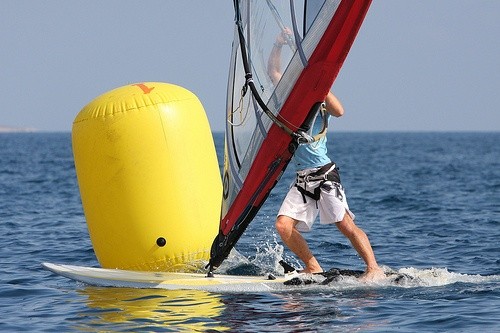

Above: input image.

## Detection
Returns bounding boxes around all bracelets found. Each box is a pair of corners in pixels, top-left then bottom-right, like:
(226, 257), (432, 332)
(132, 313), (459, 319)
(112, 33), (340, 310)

(272, 40), (283, 49)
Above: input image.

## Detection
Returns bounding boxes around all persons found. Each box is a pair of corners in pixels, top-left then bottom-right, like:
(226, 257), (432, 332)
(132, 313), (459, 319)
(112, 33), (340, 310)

(266, 27), (387, 282)
